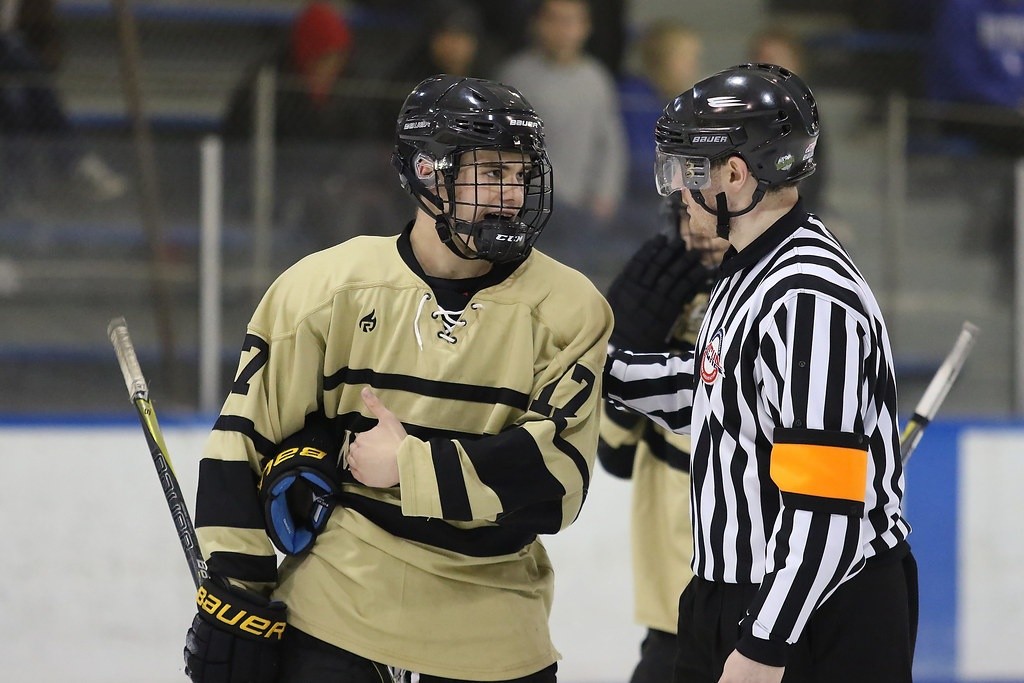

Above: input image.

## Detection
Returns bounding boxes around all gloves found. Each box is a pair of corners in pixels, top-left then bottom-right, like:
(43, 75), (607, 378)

(185, 580), (286, 682)
(259, 408), (349, 556)
(605, 233), (705, 352)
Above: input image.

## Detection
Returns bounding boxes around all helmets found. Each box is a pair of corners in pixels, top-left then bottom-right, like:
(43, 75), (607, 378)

(392, 72), (553, 264)
(653, 61), (820, 188)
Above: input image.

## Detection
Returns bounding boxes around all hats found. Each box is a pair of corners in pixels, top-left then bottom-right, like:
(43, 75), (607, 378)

(294, 4), (353, 68)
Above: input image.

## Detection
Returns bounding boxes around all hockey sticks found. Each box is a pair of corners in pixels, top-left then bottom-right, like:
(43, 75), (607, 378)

(900, 322), (983, 465)
(107, 316), (210, 594)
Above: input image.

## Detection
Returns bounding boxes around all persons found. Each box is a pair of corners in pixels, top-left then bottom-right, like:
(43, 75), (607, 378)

(215, 0), (396, 141)
(379, 0), (486, 140)
(750, 28), (855, 252)
(182, 73), (617, 683)
(600, 64), (923, 683)
(931, 0), (1024, 158)
(491, 0), (658, 252)
(609, 18), (708, 207)
